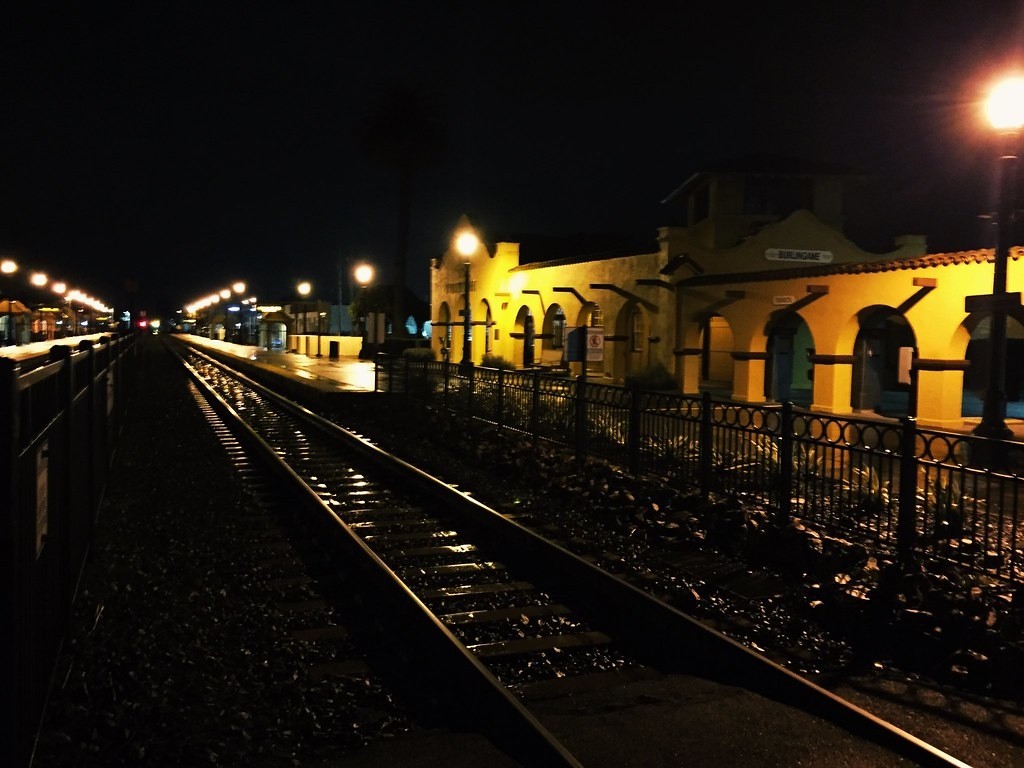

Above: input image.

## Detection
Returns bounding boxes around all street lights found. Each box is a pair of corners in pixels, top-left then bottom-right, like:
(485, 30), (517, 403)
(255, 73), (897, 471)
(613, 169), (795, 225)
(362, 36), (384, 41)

(455, 233), (480, 377)
(968, 60), (1024, 467)
(30, 272), (48, 321)
(0, 258), (20, 346)
(353, 263), (376, 338)
